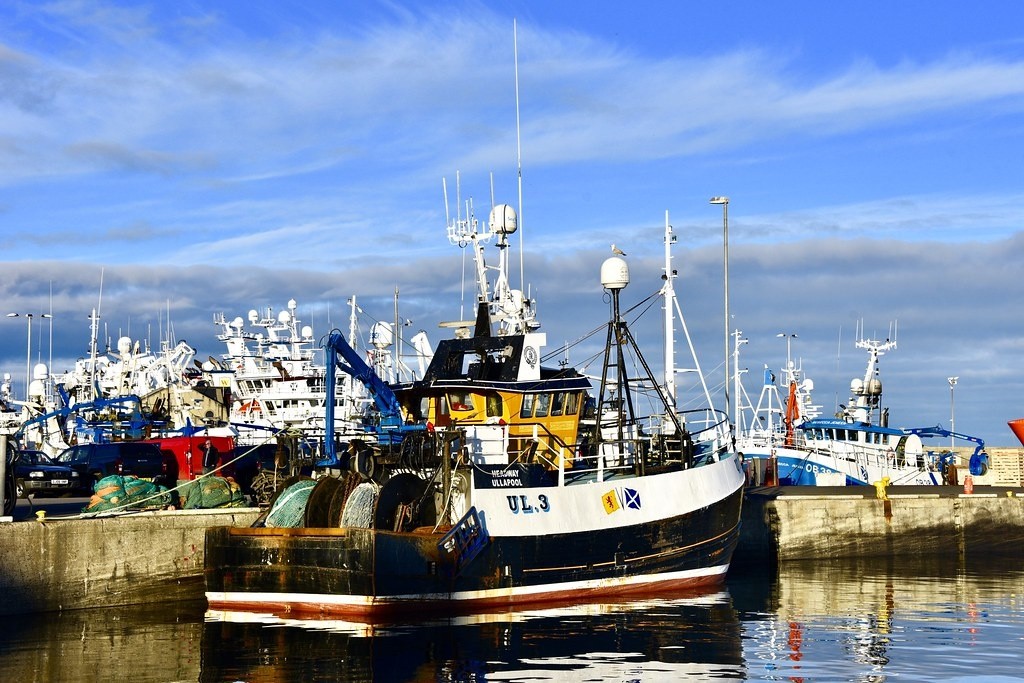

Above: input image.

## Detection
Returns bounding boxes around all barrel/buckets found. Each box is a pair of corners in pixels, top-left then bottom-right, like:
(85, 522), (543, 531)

(964, 475), (973, 494)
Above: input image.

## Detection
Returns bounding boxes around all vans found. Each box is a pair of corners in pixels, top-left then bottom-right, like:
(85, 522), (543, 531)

(49, 441), (170, 493)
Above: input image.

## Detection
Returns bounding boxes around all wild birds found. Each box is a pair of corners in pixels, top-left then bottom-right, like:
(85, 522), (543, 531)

(610, 243), (627, 257)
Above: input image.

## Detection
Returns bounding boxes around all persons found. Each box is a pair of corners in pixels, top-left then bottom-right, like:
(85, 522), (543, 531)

(197, 440), (220, 478)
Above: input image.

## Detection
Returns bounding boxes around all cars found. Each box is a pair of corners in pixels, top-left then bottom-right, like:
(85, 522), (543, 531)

(7, 450), (82, 498)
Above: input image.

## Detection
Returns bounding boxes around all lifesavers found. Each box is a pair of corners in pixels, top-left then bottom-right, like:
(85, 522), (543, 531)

(886, 448), (895, 462)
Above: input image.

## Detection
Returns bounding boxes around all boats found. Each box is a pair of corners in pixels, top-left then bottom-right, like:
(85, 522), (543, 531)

(0, 268), (436, 482)
(686, 314), (991, 491)
(200, 19), (754, 635)
